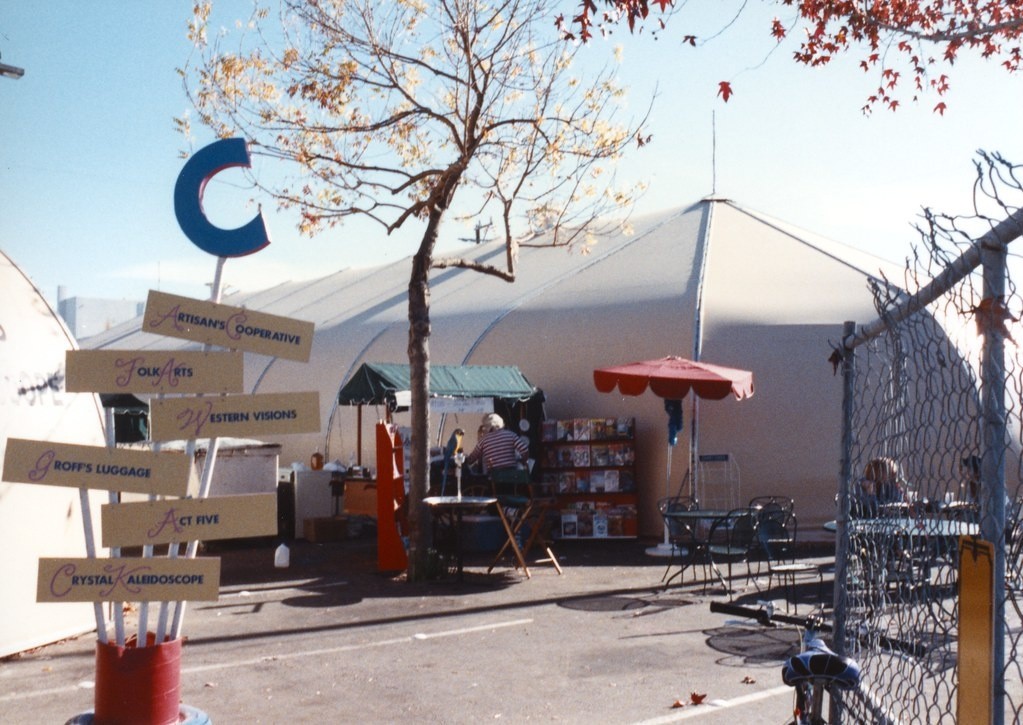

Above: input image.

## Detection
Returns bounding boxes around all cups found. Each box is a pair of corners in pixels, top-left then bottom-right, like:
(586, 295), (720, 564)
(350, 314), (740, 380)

(945, 492), (954, 504)
(907, 491), (918, 504)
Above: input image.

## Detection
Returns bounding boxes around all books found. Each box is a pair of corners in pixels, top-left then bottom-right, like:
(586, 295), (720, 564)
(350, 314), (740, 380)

(545, 417), (638, 536)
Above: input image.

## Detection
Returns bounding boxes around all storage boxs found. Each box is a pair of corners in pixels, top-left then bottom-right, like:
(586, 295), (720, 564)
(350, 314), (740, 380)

(303, 515), (348, 544)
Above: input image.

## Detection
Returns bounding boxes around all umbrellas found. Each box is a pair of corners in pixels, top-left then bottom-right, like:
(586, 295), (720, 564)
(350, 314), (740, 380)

(592, 356), (753, 554)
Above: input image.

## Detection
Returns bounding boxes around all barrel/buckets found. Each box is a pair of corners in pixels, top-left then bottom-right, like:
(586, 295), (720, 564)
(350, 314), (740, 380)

(94, 630), (182, 725)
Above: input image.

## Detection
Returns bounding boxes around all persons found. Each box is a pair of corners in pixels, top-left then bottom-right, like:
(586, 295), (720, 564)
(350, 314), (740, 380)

(936, 454), (1012, 556)
(465, 412), (542, 556)
(853, 457), (911, 566)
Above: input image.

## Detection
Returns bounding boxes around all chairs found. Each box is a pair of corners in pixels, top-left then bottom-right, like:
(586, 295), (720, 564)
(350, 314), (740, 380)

(487, 457), (562, 580)
(656, 491), (859, 616)
(452, 485), (493, 516)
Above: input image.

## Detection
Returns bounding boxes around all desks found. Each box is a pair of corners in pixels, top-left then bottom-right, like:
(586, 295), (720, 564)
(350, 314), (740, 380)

(826, 499), (981, 635)
(422, 495), (498, 591)
(662, 510), (758, 596)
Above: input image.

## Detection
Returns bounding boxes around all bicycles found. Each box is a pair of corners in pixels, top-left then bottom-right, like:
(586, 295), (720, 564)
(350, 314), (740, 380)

(709, 599), (927, 725)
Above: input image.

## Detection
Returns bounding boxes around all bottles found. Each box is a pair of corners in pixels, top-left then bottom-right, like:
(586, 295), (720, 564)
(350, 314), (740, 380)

(311, 447), (323, 471)
(274, 542), (290, 568)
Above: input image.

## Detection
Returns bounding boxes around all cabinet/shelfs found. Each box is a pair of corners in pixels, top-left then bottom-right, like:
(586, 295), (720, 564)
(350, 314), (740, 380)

(536, 416), (640, 540)
